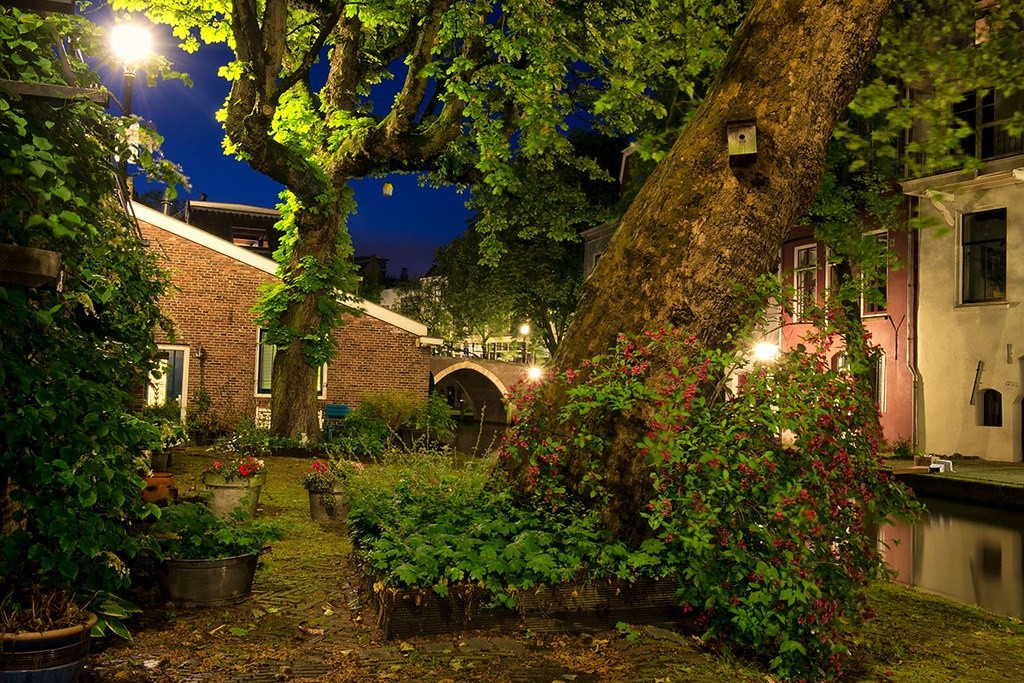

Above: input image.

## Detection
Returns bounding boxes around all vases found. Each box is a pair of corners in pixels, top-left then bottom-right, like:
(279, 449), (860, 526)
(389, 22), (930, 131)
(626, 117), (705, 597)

(307, 485), (352, 525)
(203, 472), (266, 520)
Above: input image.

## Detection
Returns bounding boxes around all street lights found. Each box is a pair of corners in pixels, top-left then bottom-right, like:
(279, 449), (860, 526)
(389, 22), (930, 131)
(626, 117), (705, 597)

(107, 23), (153, 201)
(520, 324), (529, 364)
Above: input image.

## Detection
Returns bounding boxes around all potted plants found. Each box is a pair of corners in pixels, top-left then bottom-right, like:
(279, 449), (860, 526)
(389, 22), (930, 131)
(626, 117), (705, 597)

(0, 490), (296, 683)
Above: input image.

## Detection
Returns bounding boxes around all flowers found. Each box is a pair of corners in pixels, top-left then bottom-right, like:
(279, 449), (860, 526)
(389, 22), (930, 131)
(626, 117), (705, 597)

(204, 453), (266, 484)
(304, 460), (364, 516)
